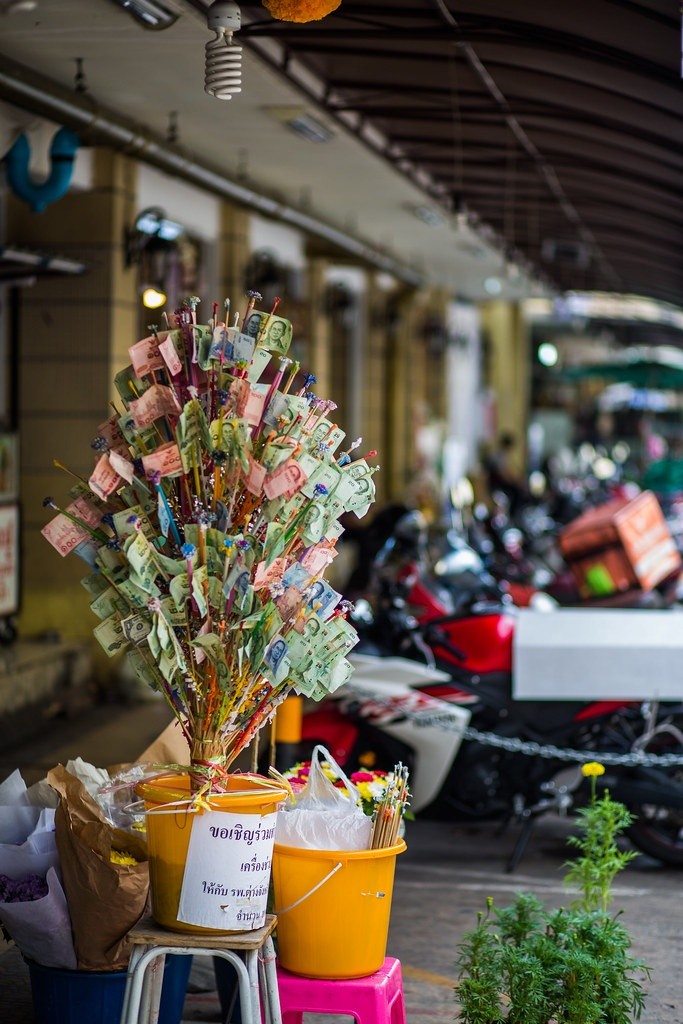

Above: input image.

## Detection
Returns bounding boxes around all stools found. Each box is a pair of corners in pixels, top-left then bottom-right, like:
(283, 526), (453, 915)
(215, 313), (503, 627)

(259, 956), (406, 1024)
(123, 911), (283, 1024)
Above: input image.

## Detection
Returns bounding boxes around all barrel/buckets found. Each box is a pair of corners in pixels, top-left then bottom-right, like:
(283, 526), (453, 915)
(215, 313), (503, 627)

(271, 838), (407, 981)
(120, 775), (288, 936)
(17, 950), (192, 1023)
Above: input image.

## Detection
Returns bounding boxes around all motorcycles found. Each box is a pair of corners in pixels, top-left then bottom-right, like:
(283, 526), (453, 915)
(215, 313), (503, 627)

(267, 427), (682, 876)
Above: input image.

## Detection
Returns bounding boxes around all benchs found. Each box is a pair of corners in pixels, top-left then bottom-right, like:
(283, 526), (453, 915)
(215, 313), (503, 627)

(276, 701), (359, 775)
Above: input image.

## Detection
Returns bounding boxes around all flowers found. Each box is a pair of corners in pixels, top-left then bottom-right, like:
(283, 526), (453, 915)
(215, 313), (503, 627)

(564, 763), (640, 912)
(276, 751), (423, 834)
